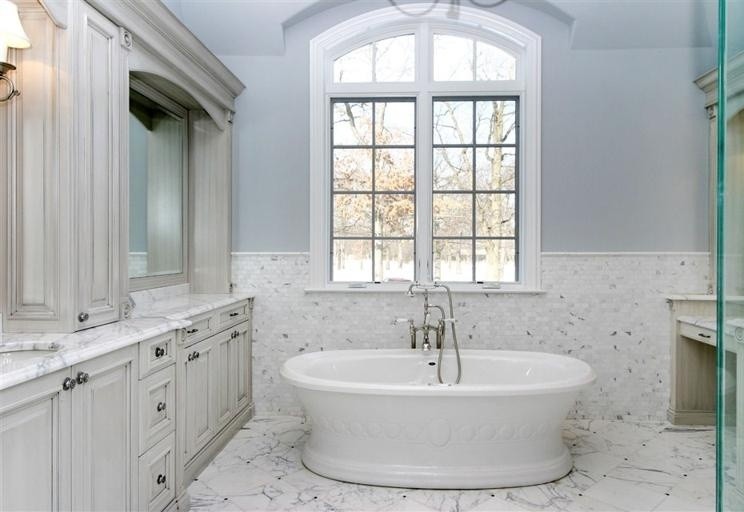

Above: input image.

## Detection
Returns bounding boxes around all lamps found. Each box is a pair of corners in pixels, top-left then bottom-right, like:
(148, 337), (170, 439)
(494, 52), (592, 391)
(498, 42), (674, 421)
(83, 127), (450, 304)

(1, 1), (33, 102)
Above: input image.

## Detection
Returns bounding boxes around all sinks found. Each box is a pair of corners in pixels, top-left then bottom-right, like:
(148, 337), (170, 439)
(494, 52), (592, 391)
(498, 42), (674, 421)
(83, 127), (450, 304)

(0, 343), (63, 374)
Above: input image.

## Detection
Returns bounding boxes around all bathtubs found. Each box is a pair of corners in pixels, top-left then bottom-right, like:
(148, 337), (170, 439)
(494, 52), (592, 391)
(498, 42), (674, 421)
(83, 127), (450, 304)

(278, 350), (597, 491)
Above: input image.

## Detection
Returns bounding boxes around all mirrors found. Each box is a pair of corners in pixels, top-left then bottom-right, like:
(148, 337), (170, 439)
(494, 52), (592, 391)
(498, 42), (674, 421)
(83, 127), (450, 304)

(128, 84), (186, 278)
